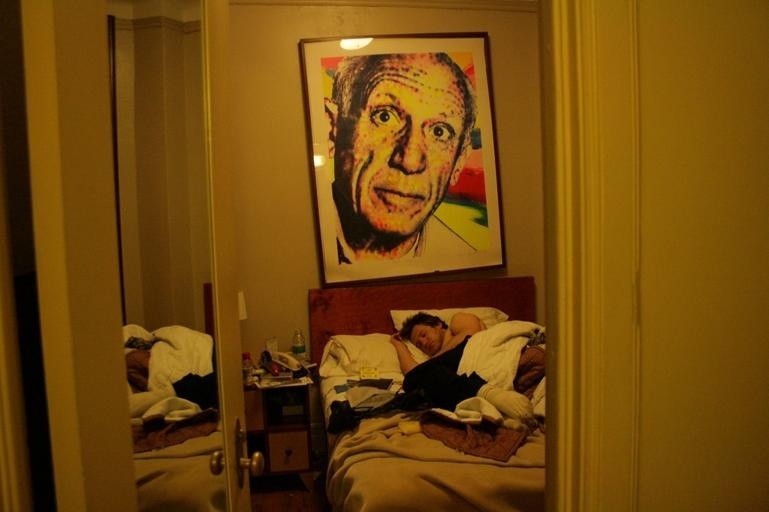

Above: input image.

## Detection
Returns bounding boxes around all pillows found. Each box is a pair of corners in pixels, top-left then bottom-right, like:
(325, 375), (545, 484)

(390, 306), (510, 365)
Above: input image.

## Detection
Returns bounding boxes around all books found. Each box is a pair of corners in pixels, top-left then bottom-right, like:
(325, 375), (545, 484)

(349, 376), (394, 391)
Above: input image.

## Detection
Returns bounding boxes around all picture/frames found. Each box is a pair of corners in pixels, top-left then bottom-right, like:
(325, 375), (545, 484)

(298, 31), (507, 290)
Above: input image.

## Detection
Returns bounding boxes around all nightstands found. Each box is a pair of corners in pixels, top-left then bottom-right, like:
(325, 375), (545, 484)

(244, 372), (325, 489)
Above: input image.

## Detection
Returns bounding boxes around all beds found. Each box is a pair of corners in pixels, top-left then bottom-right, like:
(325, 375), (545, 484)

(304, 278), (549, 512)
(121, 283), (232, 512)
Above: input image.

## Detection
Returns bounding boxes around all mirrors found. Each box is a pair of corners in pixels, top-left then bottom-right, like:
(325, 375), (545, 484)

(18, 0), (251, 512)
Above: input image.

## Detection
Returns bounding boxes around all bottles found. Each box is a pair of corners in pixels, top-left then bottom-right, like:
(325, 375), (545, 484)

(241, 352), (254, 391)
(291, 329), (306, 360)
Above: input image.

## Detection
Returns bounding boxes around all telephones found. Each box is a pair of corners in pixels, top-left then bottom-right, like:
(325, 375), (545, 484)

(271, 351), (307, 371)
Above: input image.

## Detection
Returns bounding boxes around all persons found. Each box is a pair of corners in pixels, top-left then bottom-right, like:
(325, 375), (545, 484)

(390, 311), (489, 375)
(322, 53), (478, 266)
